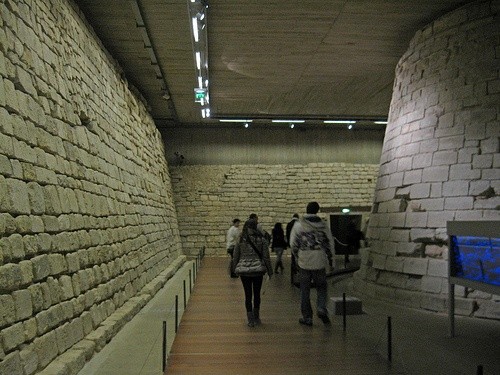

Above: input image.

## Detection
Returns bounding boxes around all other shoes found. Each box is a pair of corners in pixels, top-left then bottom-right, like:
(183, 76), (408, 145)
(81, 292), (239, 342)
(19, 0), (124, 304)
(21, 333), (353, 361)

(254, 317), (261, 322)
(317, 312), (331, 325)
(248, 322), (253, 326)
(274, 271), (279, 273)
(299, 318), (314, 326)
(281, 267), (285, 270)
(230, 274), (239, 278)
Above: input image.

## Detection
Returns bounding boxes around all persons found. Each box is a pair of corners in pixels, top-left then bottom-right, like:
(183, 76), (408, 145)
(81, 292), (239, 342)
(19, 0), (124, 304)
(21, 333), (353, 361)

(290, 201), (336, 328)
(230, 219), (274, 327)
(226, 213), (370, 278)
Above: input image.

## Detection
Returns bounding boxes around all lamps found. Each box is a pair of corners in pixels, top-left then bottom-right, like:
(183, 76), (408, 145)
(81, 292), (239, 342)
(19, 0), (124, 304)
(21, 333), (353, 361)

(186, 0), (211, 119)
